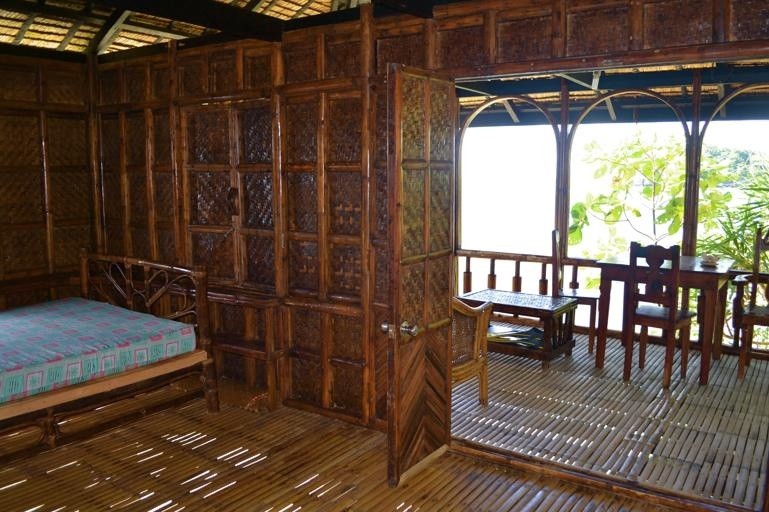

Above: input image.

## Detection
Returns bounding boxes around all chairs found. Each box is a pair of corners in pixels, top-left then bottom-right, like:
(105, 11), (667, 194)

(450, 297), (492, 407)
(552, 230), (599, 354)
(733, 228), (769, 380)
(625, 241), (697, 389)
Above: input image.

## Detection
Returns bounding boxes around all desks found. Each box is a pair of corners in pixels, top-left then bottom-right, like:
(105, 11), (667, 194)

(593, 252), (736, 388)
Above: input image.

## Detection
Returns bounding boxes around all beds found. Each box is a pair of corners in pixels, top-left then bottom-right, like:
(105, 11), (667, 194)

(1, 250), (221, 457)
